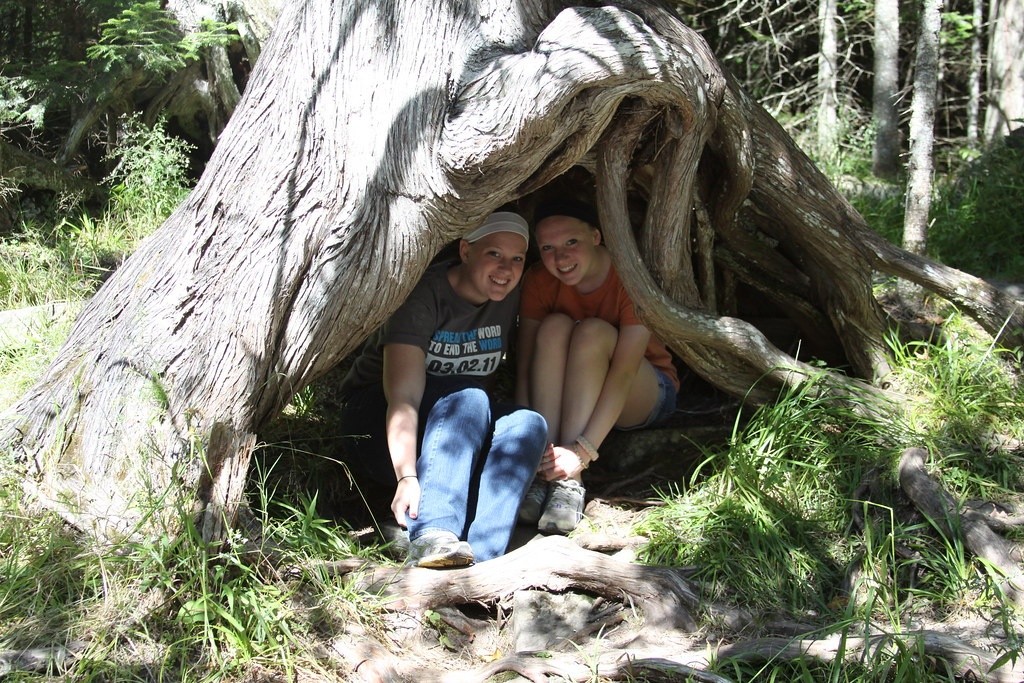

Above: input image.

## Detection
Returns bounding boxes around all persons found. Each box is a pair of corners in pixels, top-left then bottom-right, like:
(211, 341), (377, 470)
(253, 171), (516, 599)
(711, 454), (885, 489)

(511, 197), (682, 536)
(335, 202), (551, 571)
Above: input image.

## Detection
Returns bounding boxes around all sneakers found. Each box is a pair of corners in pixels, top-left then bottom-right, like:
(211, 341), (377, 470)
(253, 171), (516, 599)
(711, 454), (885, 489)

(408, 530), (474, 568)
(517, 475), (586, 535)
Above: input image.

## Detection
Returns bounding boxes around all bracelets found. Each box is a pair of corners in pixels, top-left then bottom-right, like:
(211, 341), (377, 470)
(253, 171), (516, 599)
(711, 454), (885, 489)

(397, 473), (417, 483)
(573, 442), (590, 469)
(576, 435), (599, 462)
